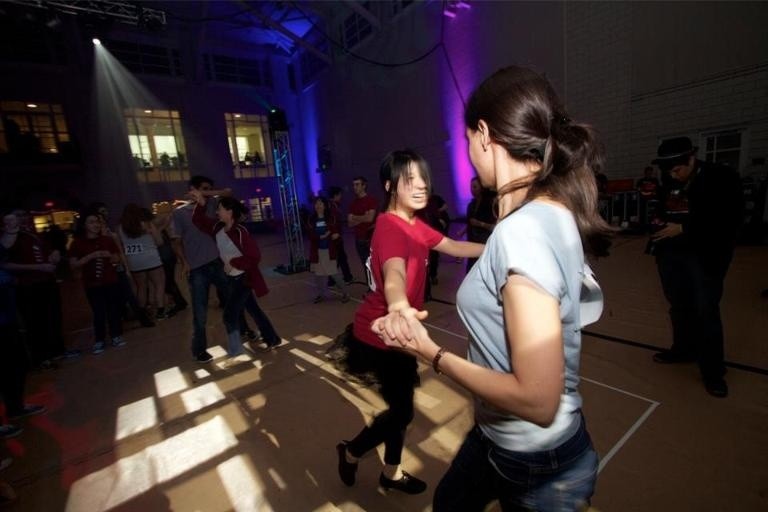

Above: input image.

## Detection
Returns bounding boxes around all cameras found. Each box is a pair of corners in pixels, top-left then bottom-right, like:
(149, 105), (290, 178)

(95, 251), (105, 257)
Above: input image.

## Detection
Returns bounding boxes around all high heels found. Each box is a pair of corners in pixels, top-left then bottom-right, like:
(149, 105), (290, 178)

(335, 438), (360, 488)
(379, 466), (428, 495)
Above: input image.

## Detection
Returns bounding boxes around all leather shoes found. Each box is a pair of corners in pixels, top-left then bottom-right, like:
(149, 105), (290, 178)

(702, 377), (728, 398)
(652, 351), (667, 364)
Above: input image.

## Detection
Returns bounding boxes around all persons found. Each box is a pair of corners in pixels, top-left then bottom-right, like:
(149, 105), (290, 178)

(1, 203), (190, 441)
(306, 176), (382, 303)
(169, 176), (281, 364)
(636, 138), (742, 399)
(413, 173), (611, 310)
(366, 64), (620, 512)
(337, 148), (497, 497)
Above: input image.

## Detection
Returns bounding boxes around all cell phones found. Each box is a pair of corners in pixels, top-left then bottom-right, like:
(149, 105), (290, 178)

(647, 224), (668, 234)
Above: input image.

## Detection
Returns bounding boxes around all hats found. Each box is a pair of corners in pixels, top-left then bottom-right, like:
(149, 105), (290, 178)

(650, 136), (698, 164)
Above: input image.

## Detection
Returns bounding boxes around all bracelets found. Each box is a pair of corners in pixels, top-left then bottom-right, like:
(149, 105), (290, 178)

(432, 346), (450, 376)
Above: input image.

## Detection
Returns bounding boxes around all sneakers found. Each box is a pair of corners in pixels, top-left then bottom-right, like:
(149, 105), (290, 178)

(8, 403), (47, 420)
(39, 360), (58, 370)
(242, 328), (257, 342)
(51, 348), (81, 361)
(0, 424), (24, 440)
(111, 336), (127, 347)
(92, 341), (104, 354)
(191, 350), (213, 363)
